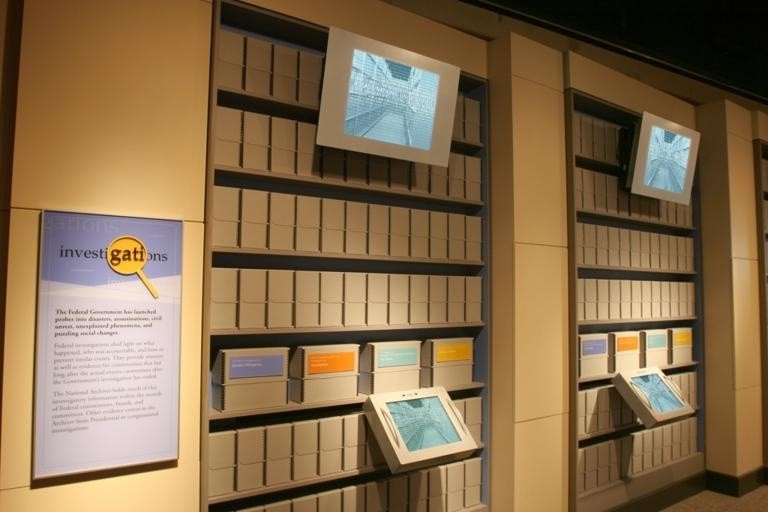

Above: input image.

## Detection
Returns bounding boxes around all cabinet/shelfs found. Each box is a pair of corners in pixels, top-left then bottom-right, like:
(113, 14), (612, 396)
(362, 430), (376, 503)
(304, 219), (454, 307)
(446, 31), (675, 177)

(563, 48), (709, 512)
(197, 0), (491, 510)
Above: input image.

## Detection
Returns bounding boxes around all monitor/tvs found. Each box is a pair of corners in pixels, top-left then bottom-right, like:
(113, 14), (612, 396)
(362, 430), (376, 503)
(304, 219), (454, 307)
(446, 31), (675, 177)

(363, 386), (478, 474)
(315, 25), (461, 167)
(611, 365), (695, 429)
(626, 111), (701, 206)
